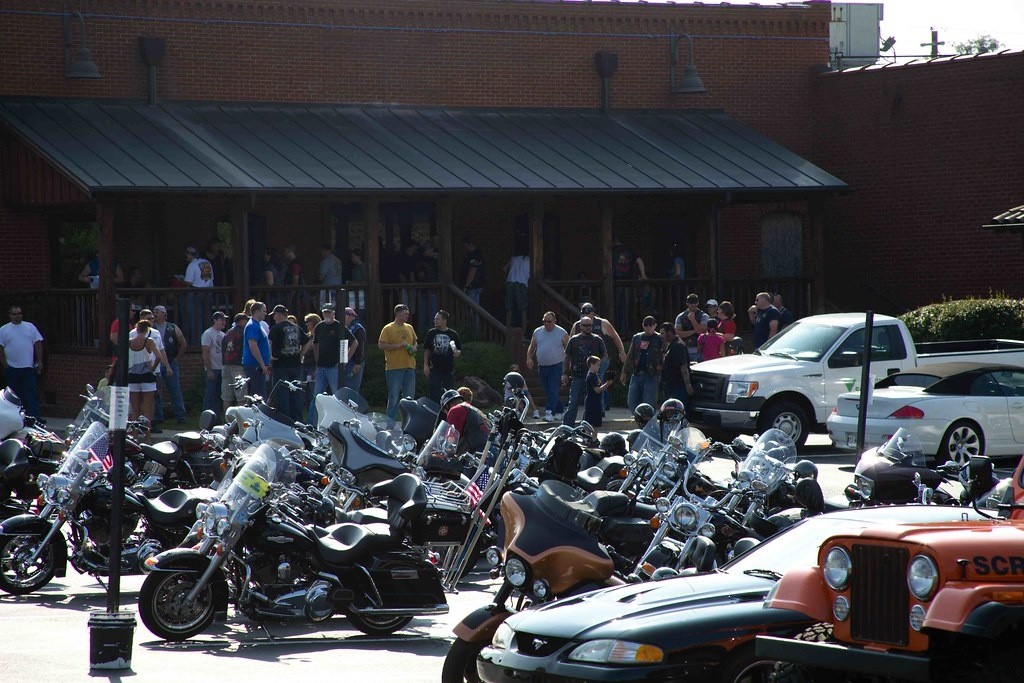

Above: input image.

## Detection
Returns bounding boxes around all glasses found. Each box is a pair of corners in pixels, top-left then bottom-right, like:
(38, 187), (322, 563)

(757, 297), (761, 301)
(643, 324), (653, 327)
(687, 301), (698, 304)
(543, 319), (555, 323)
(581, 323), (593, 327)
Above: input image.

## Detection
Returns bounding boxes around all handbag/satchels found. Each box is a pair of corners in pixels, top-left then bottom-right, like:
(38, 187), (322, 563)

(697, 345), (703, 362)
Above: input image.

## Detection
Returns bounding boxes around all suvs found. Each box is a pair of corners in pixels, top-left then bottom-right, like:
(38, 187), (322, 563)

(751, 461), (1024, 683)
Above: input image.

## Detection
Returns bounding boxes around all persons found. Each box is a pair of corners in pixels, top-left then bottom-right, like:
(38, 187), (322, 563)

(201, 299), (366, 432)
(501, 232), (694, 343)
(377, 303), (419, 430)
(0, 306), (47, 424)
(80, 234), (486, 348)
(96, 300), (187, 446)
(433, 387), (492, 458)
(423, 309), (462, 404)
(504, 292), (793, 446)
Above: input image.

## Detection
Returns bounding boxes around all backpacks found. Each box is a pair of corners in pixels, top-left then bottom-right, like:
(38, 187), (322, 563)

(460, 404), (493, 448)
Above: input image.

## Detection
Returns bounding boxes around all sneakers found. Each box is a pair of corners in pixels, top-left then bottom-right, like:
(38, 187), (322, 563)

(554, 413), (563, 420)
(533, 410), (539, 418)
(544, 410), (553, 421)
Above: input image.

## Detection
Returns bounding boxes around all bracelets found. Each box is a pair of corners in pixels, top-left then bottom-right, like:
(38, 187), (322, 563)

(462, 284), (469, 289)
(165, 365), (171, 370)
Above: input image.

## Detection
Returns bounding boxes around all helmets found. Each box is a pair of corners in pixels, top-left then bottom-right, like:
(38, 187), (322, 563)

(598, 431), (626, 453)
(633, 403), (656, 423)
(657, 398), (686, 423)
(441, 388), (463, 405)
(504, 372), (525, 391)
(626, 429), (644, 446)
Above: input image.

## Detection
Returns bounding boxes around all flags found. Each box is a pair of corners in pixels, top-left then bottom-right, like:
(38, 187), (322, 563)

(84, 433), (114, 478)
(463, 464), (495, 526)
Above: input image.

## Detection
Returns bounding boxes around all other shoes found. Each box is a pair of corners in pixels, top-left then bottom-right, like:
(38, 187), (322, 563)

(151, 426), (162, 433)
(37, 418), (47, 425)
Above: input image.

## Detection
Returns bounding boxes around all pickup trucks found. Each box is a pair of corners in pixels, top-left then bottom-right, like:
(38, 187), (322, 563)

(689, 312), (1024, 461)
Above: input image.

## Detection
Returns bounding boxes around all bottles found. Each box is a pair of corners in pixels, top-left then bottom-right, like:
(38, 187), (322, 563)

(405, 344), (416, 356)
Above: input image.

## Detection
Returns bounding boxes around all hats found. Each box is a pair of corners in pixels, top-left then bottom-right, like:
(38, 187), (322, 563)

(720, 301), (734, 315)
(152, 306), (167, 313)
(268, 305), (289, 317)
(345, 308), (357, 318)
(211, 311), (230, 319)
(322, 303), (335, 313)
(706, 299), (719, 306)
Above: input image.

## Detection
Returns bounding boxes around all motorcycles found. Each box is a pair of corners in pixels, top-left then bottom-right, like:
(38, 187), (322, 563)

(1, 374), (1014, 683)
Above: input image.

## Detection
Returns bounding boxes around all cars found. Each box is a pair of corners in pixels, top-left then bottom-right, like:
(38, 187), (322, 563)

(476, 504), (1001, 683)
(825, 361), (1024, 468)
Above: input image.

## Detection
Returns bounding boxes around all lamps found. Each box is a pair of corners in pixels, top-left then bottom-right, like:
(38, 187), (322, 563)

(670, 26), (709, 95)
(63, 7), (103, 79)
